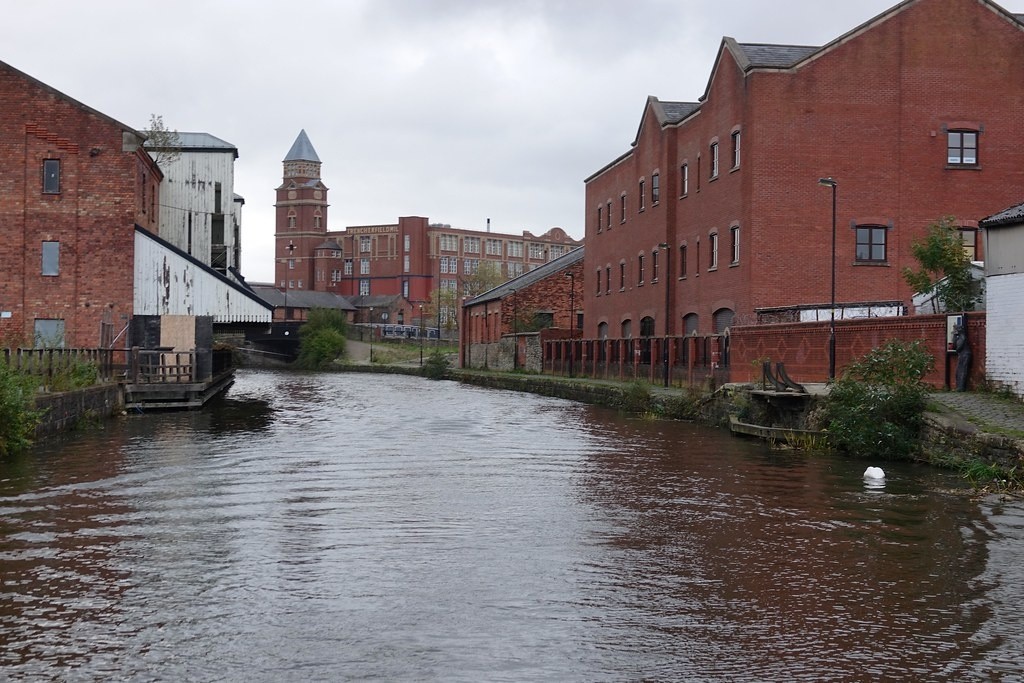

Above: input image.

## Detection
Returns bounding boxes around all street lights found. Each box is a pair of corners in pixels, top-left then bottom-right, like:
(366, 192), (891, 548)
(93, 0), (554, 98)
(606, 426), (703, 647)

(360, 293), (363, 341)
(819, 177), (838, 386)
(509, 288), (517, 369)
(419, 304), (423, 365)
(564, 272), (573, 378)
(369, 306), (373, 362)
